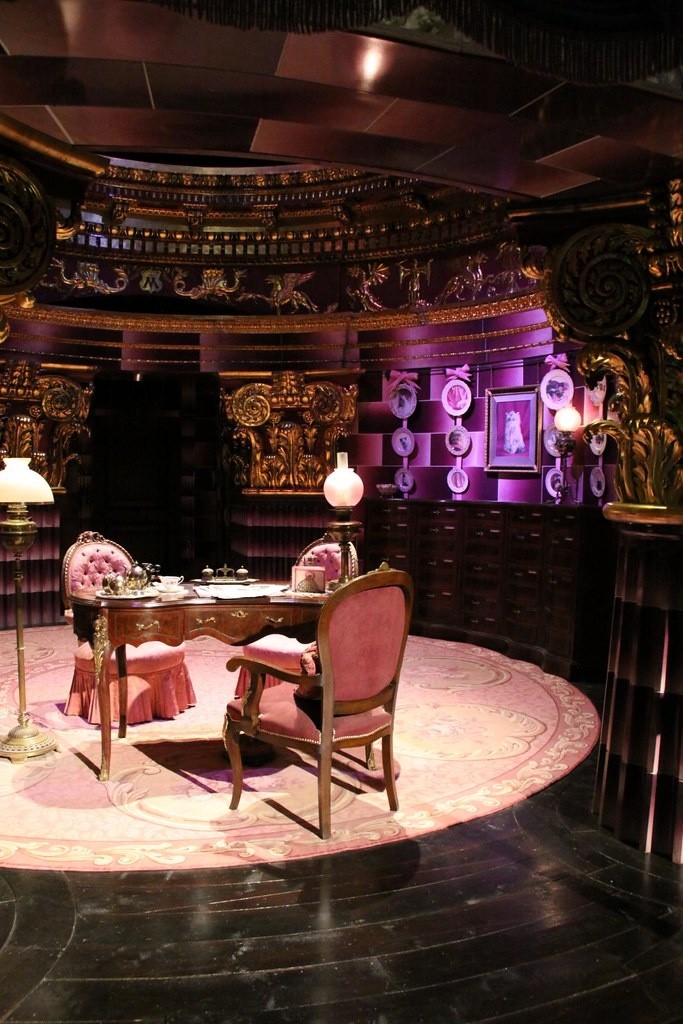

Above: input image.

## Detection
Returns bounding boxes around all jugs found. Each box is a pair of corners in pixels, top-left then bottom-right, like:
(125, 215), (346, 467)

(158, 575), (184, 588)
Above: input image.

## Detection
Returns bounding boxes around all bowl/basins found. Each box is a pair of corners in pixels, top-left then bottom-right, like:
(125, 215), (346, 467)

(376, 483), (399, 494)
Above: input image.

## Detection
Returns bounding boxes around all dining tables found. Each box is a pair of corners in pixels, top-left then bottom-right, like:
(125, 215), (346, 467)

(63, 578), (378, 783)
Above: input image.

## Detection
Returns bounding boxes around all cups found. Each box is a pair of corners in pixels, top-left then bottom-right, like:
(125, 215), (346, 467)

(202, 565), (213, 580)
(236, 566), (248, 580)
(102, 571), (115, 595)
(110, 574), (125, 595)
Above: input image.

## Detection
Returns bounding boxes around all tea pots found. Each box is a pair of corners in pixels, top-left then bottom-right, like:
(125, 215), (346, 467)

(123, 561), (151, 590)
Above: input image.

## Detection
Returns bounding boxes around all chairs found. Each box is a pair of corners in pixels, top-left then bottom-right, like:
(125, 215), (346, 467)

(232, 537), (360, 702)
(60, 531), (197, 728)
(221, 562), (416, 840)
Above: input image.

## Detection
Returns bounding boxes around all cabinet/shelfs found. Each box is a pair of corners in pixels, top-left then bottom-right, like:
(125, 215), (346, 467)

(366, 495), (617, 681)
(229, 498), (363, 581)
(0, 494), (72, 629)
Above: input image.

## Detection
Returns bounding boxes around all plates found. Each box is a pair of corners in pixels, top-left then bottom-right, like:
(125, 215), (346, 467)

(96, 590), (161, 599)
(155, 586), (184, 592)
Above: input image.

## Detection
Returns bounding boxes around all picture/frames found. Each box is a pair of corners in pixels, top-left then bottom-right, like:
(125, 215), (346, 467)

(386, 367), (607, 497)
(484, 384), (545, 477)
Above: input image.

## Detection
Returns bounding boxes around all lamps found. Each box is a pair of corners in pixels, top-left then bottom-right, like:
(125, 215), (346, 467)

(554, 406), (582, 506)
(323, 451), (364, 591)
(0, 456), (55, 764)
